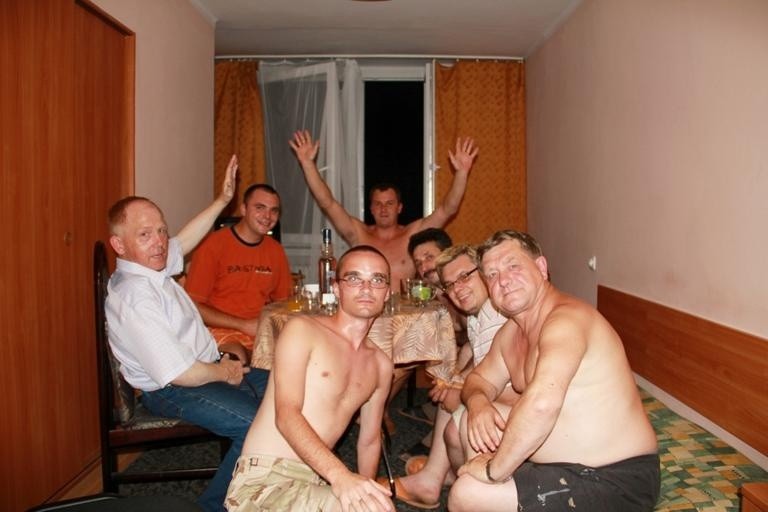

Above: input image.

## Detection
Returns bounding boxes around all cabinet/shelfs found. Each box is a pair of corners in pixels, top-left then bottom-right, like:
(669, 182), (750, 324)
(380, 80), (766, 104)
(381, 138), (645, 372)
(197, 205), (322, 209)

(0, 1), (134, 512)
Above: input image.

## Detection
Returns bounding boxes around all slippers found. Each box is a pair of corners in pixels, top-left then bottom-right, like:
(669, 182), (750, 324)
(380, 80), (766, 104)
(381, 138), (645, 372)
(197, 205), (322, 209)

(398, 441), (431, 462)
(405, 456), (455, 487)
(397, 405), (435, 427)
(376, 475), (441, 510)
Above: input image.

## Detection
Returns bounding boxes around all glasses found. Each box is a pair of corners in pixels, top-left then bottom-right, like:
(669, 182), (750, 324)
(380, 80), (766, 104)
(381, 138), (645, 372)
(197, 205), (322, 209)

(442, 266), (478, 294)
(336, 275), (391, 289)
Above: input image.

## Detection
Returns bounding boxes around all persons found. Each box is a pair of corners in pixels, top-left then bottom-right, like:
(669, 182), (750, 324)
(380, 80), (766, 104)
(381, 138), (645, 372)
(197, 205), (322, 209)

(448, 229), (661, 512)
(396, 227), (468, 462)
(224, 244), (399, 512)
(184, 183), (292, 354)
(379, 242), (510, 511)
(103, 154), (270, 512)
(288, 129), (479, 294)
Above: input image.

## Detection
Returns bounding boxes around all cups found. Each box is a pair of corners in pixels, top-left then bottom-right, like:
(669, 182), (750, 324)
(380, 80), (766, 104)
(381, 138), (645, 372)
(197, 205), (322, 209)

(285, 286), (302, 312)
(399, 276), (416, 307)
(410, 280), (438, 307)
(381, 293), (398, 317)
(302, 289), (318, 316)
(320, 295), (336, 317)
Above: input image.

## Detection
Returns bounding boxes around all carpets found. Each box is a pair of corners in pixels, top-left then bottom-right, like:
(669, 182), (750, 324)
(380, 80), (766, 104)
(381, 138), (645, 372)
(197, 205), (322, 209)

(100, 387), (451, 511)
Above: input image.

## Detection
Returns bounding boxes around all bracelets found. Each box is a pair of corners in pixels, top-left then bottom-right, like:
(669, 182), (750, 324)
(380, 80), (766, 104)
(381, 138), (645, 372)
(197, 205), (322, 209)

(486, 459), (512, 484)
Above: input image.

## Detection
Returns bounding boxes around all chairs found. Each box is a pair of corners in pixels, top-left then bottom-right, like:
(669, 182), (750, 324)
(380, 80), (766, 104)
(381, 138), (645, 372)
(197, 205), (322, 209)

(92, 241), (232, 494)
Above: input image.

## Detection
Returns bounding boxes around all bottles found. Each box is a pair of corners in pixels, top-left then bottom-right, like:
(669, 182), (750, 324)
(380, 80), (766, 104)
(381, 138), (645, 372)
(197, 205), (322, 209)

(318, 228), (338, 305)
